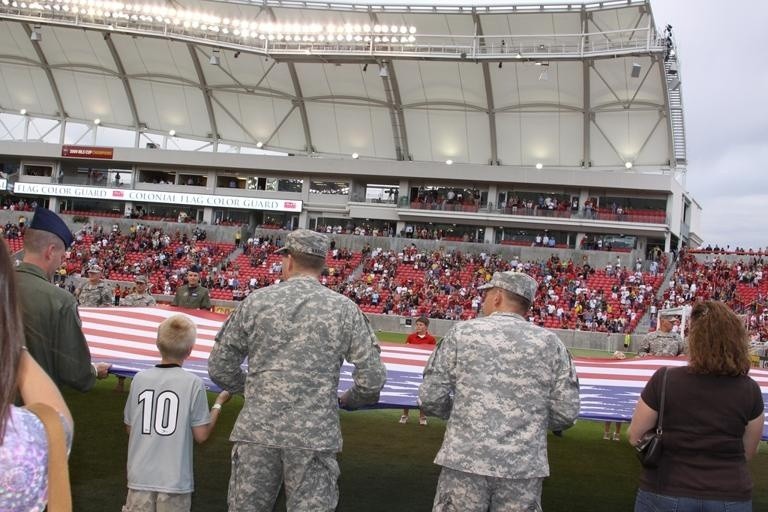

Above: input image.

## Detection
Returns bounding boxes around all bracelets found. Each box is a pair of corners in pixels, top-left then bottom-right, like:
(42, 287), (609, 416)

(211, 403), (222, 410)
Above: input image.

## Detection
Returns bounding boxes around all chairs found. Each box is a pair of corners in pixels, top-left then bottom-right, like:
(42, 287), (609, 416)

(727, 260), (768, 343)
(319, 247), (363, 286)
(3, 234), (24, 254)
(209, 245), (286, 301)
(64, 233), (237, 294)
(612, 246), (632, 253)
(62, 209), (124, 218)
(533, 292), (577, 330)
(587, 269), (665, 334)
(501, 239), (567, 249)
(220, 221), (278, 228)
(359, 261), (477, 320)
(142, 214), (199, 224)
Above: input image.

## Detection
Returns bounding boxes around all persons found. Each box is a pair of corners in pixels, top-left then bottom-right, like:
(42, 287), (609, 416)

(627, 303), (764, 512)
(650, 303), (657, 333)
(309, 221), (395, 238)
(0, 199), (38, 240)
(123, 314), (233, 512)
(657, 303), (693, 309)
(86, 167), (106, 186)
(581, 235), (612, 253)
(732, 309), (768, 342)
(535, 234), (556, 248)
(143, 178), (174, 184)
(208, 231), (387, 512)
(170, 273), (188, 294)
(604, 351), (624, 440)
(112, 206), (293, 231)
(493, 252), (524, 272)
(419, 272), (581, 512)
(405, 224), (485, 243)
(400, 318), (437, 425)
(310, 188), (349, 196)
(410, 185), (480, 212)
(55, 275), (75, 298)
(387, 188), (399, 204)
(12, 204), (110, 512)
(57, 168), (64, 183)
(525, 253), (668, 335)
(170, 266), (211, 313)
(209, 232), (286, 302)
(115, 284), (121, 306)
(52, 221), (208, 274)
(499, 193), (623, 222)
(189, 178), (193, 184)
(230, 180), (236, 187)
(649, 243), (768, 309)
(0, 237), (75, 512)
(319, 238), (493, 320)
(112, 275), (159, 393)
(160, 274), (169, 294)
(638, 309), (688, 358)
(77, 265), (112, 307)
(114, 171), (121, 187)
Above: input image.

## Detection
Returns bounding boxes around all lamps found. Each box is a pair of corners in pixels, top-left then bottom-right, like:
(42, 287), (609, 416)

(379, 68), (386, 77)
(539, 72), (548, 79)
(210, 56), (219, 66)
(632, 61), (640, 78)
(28, 31), (42, 40)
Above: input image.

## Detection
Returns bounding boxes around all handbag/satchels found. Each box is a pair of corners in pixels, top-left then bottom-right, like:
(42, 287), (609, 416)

(634, 365), (670, 471)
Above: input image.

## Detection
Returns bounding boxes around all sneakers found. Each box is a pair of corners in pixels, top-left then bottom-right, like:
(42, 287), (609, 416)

(419, 416), (427, 424)
(613, 432), (619, 441)
(399, 415), (407, 423)
(603, 432), (611, 441)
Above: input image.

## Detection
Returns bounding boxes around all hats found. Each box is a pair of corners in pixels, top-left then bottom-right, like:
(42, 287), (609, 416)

(659, 313), (678, 322)
(272, 228), (330, 261)
(29, 204), (76, 252)
(134, 275), (149, 286)
(476, 271), (540, 304)
(86, 265), (105, 275)
(187, 265), (204, 274)
